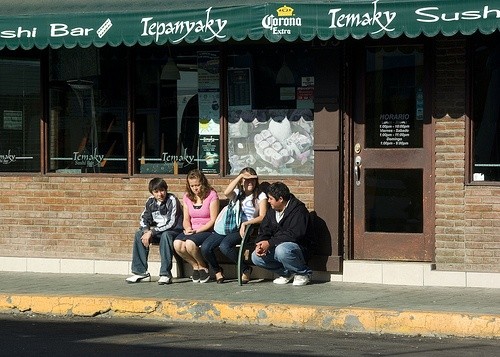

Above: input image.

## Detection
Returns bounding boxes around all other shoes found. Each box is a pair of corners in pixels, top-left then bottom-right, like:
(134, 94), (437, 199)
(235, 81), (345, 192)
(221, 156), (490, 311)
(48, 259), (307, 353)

(273, 273), (294, 284)
(192, 265), (200, 283)
(241, 266), (253, 284)
(214, 267), (224, 283)
(199, 264), (210, 283)
(292, 273), (311, 286)
(125, 273), (151, 283)
(157, 276), (173, 285)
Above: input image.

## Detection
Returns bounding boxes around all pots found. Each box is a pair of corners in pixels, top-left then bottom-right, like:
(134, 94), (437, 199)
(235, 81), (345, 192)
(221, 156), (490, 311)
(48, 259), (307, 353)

(247, 120), (314, 169)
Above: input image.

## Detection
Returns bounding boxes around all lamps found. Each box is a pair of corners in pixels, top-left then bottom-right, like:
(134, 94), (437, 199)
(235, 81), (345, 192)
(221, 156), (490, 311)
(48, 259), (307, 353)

(276, 56), (295, 84)
(160, 45), (180, 80)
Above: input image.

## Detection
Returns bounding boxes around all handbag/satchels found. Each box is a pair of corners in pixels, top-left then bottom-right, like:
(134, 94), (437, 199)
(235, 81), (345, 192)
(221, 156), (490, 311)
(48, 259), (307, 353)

(214, 189), (242, 235)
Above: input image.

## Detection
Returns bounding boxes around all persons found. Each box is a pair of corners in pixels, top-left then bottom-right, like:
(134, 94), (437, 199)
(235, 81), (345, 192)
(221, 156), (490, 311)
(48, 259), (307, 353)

(181, 95), (198, 174)
(173, 170), (220, 283)
(201, 167), (267, 283)
(125, 178), (184, 285)
(251, 182), (313, 286)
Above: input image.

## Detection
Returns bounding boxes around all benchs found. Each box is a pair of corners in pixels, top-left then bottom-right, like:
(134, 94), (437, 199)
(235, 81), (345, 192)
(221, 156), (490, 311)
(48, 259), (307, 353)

(152, 199), (253, 285)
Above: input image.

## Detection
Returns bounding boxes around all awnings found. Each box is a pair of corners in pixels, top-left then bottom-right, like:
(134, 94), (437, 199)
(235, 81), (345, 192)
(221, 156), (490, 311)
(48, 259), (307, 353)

(0, 0), (500, 51)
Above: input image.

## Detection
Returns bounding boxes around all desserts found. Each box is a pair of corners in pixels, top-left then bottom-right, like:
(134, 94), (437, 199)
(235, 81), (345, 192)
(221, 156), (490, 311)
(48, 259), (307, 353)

(254, 130), (290, 167)
